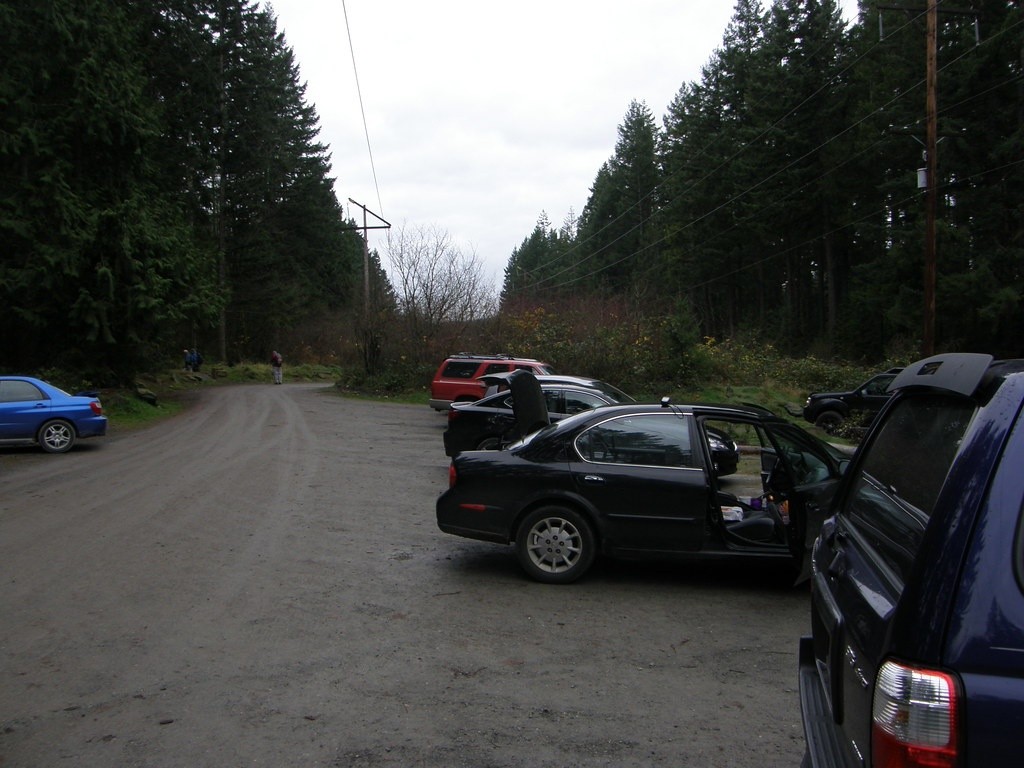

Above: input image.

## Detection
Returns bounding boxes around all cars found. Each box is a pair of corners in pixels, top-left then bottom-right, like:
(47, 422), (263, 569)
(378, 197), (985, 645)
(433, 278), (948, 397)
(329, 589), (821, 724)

(435, 395), (856, 586)
(477, 373), (638, 406)
(441, 384), (740, 479)
(0, 377), (108, 455)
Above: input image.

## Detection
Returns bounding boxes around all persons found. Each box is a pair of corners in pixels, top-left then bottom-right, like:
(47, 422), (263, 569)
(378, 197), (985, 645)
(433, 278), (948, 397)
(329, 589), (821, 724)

(184, 349), (202, 372)
(272, 351), (282, 384)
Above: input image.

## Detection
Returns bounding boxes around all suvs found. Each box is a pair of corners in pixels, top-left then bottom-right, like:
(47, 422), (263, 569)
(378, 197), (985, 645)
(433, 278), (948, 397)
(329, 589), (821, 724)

(428, 351), (561, 411)
(799, 351), (1024, 768)
(802, 366), (905, 436)
